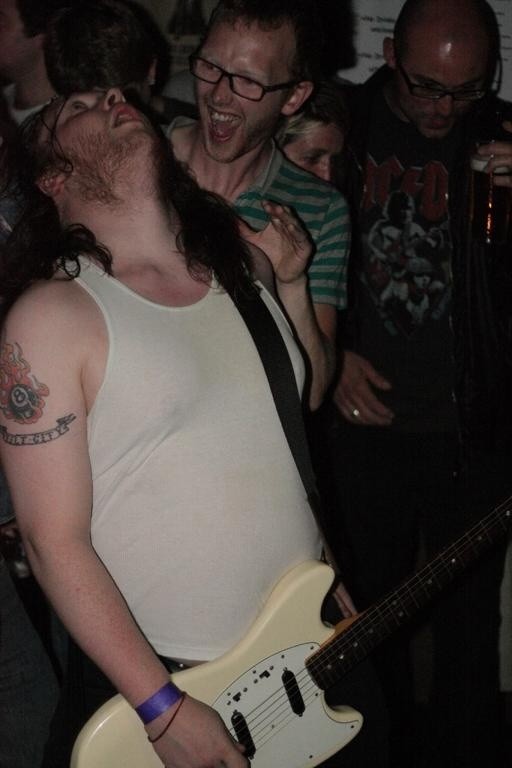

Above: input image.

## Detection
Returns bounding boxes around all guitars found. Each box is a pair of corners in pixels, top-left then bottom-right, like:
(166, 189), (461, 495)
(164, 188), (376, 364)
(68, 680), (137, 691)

(69, 497), (512, 768)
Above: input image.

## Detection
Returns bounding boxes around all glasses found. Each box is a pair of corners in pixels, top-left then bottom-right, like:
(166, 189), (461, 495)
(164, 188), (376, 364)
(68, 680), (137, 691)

(186, 52), (296, 102)
(391, 47), (505, 106)
